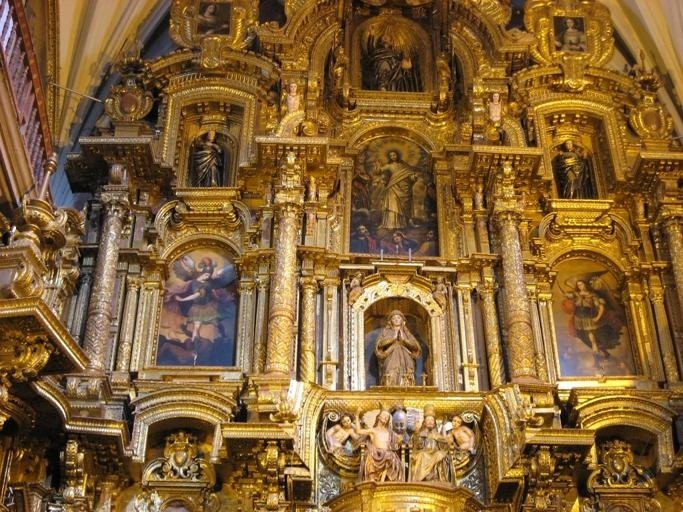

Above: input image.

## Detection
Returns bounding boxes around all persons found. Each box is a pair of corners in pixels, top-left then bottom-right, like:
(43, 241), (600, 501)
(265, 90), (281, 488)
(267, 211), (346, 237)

(556, 274), (618, 372)
(484, 91), (508, 146)
(162, 250), (238, 367)
(198, 2), (229, 39)
(555, 139), (591, 200)
(375, 309), (421, 387)
(188, 130), (225, 189)
(322, 401), (480, 487)
(553, 16), (587, 54)
(276, 82), (304, 140)
(350, 149), (439, 256)
(364, 32), (413, 94)
